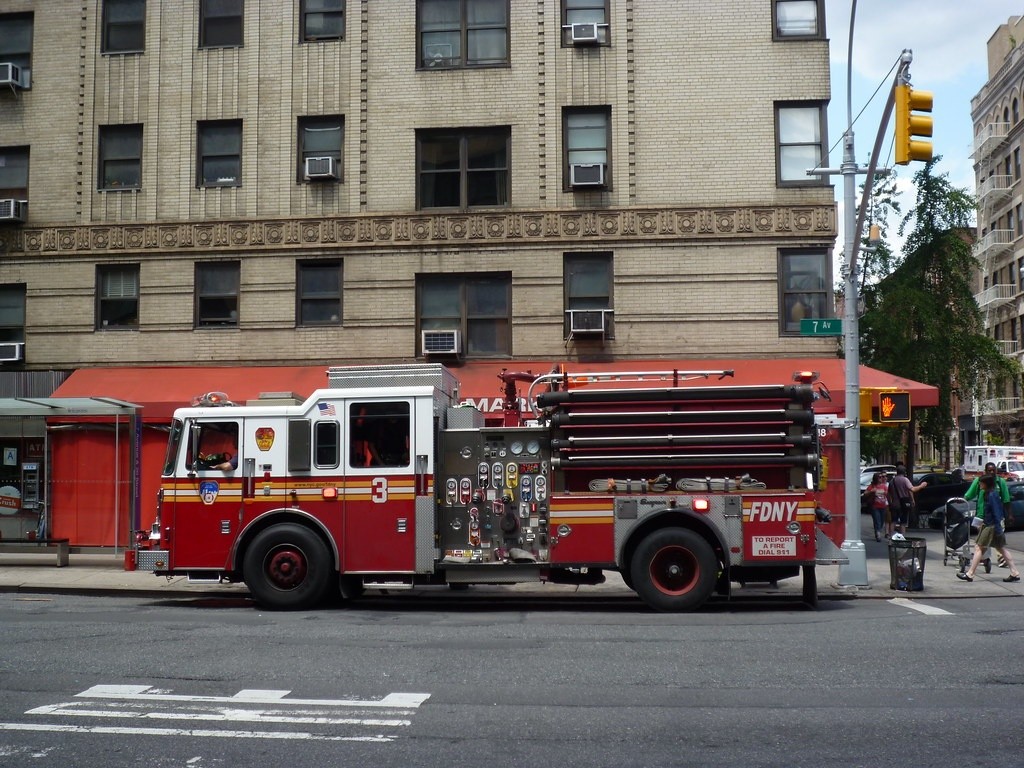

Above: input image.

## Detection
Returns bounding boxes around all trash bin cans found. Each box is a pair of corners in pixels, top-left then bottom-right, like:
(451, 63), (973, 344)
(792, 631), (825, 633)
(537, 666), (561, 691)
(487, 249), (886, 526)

(887, 537), (927, 592)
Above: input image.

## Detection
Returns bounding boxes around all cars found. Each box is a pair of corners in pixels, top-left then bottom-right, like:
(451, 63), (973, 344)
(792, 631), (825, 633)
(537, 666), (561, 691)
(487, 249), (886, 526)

(907, 471), (966, 513)
(859, 465), (923, 510)
(927, 479), (1024, 533)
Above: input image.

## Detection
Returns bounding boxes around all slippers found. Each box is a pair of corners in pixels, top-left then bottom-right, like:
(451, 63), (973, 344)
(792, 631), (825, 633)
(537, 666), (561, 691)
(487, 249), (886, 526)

(1002, 575), (1021, 582)
(956, 572), (974, 582)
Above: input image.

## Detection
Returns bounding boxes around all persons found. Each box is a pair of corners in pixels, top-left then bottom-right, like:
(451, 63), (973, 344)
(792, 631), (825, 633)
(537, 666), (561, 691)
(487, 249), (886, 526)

(956, 475), (1020, 582)
(209, 424), (238, 471)
(963, 462), (1014, 567)
(864, 466), (927, 542)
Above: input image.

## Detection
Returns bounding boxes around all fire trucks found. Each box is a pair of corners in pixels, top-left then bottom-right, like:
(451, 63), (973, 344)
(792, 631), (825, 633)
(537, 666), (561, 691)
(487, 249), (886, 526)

(963, 446), (1024, 483)
(122, 361), (830, 614)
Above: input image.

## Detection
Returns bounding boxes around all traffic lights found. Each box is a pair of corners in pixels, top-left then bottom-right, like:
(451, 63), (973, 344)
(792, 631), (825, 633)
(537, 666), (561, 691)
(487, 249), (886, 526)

(893, 83), (938, 166)
(877, 391), (923, 425)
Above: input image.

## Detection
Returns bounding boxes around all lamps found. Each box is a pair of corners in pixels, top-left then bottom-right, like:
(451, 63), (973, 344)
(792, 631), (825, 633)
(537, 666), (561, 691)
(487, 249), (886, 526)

(0, 198), (21, 220)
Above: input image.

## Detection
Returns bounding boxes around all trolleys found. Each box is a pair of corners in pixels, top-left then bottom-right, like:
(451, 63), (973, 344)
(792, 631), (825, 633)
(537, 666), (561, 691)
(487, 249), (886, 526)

(942, 497), (994, 576)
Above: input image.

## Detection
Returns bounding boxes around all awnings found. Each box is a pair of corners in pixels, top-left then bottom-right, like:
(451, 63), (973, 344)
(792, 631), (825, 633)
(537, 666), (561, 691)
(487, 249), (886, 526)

(41, 360), (939, 422)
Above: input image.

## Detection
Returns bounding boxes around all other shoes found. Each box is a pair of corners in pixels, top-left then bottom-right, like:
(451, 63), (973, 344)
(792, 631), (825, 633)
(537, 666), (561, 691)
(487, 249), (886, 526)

(885, 534), (891, 540)
(877, 537), (881, 542)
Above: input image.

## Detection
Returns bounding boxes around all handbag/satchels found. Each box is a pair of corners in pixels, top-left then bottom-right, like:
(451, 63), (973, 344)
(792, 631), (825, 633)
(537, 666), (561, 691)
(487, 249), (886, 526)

(865, 483), (876, 507)
(901, 497), (914, 511)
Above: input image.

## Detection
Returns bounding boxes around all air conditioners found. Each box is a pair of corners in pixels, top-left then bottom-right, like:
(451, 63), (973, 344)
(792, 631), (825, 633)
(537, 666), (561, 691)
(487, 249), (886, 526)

(571, 164), (603, 184)
(0, 342), (25, 361)
(0, 62), (23, 86)
(421, 330), (461, 354)
(572, 22), (597, 40)
(570, 310), (604, 332)
(305, 157), (336, 178)
